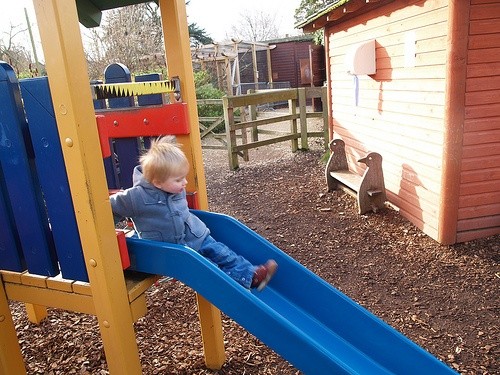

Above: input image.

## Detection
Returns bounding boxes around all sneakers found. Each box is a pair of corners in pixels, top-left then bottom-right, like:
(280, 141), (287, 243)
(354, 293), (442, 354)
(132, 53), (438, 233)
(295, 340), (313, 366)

(250, 262), (280, 291)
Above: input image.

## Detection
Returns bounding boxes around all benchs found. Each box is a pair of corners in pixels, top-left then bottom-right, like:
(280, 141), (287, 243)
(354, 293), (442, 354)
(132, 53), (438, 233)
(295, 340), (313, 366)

(324, 138), (390, 215)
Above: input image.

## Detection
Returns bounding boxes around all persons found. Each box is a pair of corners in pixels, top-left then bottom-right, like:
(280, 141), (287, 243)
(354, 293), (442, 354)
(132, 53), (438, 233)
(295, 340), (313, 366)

(109, 135), (278, 295)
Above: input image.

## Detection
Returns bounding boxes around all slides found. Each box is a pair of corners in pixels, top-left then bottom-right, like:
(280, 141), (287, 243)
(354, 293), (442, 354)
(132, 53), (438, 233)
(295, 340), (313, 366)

(121, 208), (461, 375)
